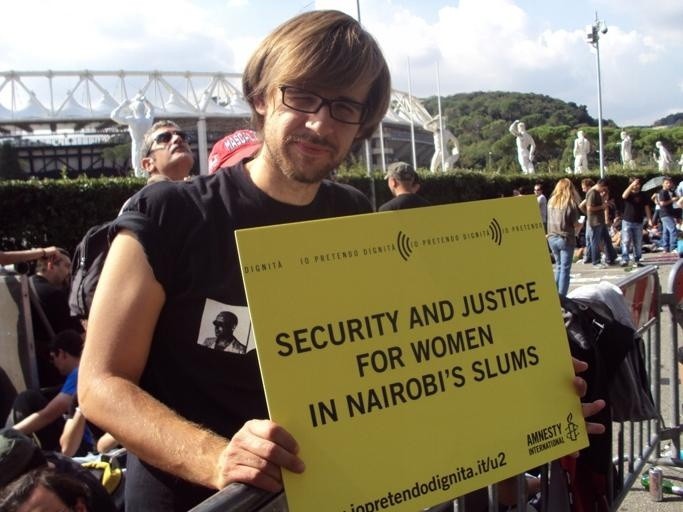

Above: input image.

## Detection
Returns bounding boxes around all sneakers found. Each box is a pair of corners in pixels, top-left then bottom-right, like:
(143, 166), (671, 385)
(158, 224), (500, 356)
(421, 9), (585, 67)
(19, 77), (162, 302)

(591, 260), (644, 268)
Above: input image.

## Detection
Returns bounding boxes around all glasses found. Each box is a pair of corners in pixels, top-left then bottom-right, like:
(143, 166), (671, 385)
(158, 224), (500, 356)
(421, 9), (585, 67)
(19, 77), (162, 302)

(278, 83), (368, 125)
(145, 130), (186, 158)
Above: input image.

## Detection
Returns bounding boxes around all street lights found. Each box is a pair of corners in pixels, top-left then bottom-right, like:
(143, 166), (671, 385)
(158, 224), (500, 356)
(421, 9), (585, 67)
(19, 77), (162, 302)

(585, 9), (610, 179)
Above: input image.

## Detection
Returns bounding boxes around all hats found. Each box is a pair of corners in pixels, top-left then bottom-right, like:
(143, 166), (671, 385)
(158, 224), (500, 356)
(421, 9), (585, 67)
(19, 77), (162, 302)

(383, 162), (413, 180)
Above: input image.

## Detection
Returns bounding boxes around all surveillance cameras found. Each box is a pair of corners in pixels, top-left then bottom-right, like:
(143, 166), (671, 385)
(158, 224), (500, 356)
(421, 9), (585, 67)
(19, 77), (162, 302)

(601, 19), (608, 33)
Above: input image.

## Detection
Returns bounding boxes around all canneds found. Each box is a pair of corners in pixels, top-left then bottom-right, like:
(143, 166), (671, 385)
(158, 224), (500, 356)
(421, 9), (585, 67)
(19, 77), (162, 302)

(649, 467), (662, 501)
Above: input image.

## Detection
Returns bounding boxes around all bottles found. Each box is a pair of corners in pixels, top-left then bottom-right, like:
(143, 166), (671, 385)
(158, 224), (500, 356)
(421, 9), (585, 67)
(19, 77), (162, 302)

(641, 473), (682, 495)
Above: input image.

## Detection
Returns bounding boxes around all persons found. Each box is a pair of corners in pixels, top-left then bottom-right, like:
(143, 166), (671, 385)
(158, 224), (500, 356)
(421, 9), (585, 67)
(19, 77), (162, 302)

(508, 118), (538, 174)
(202, 310), (246, 356)
(652, 140), (674, 173)
(617, 129), (635, 169)
(375, 160), (436, 215)
(109, 89), (154, 177)
(74, 7), (607, 512)
(114, 120), (196, 225)
(423, 112), (460, 173)
(572, 130), (590, 174)
(1, 244), (81, 406)
(497, 174), (683, 294)
(9, 308), (127, 462)
(2, 427), (117, 512)
